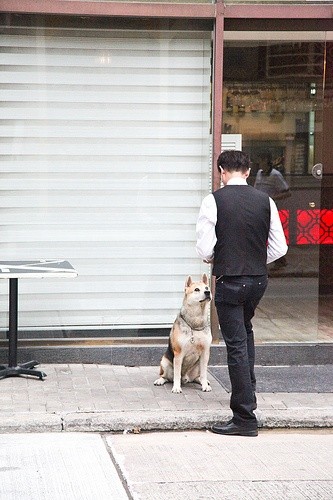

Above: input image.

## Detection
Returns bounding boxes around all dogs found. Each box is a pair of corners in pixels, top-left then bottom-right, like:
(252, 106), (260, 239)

(152, 270), (214, 395)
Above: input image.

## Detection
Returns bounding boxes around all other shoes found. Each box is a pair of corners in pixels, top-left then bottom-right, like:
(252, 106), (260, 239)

(271, 263), (287, 272)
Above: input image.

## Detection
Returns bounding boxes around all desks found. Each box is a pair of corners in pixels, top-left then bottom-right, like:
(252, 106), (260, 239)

(0, 257), (78, 380)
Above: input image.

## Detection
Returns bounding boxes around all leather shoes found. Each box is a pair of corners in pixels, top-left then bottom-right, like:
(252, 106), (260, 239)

(213, 420), (259, 435)
(251, 394), (257, 410)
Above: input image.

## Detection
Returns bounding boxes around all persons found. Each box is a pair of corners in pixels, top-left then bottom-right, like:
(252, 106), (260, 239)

(254, 152), (292, 267)
(194, 149), (289, 437)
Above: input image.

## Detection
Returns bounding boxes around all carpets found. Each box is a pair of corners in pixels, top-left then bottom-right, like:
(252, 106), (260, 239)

(206, 364), (333, 394)
